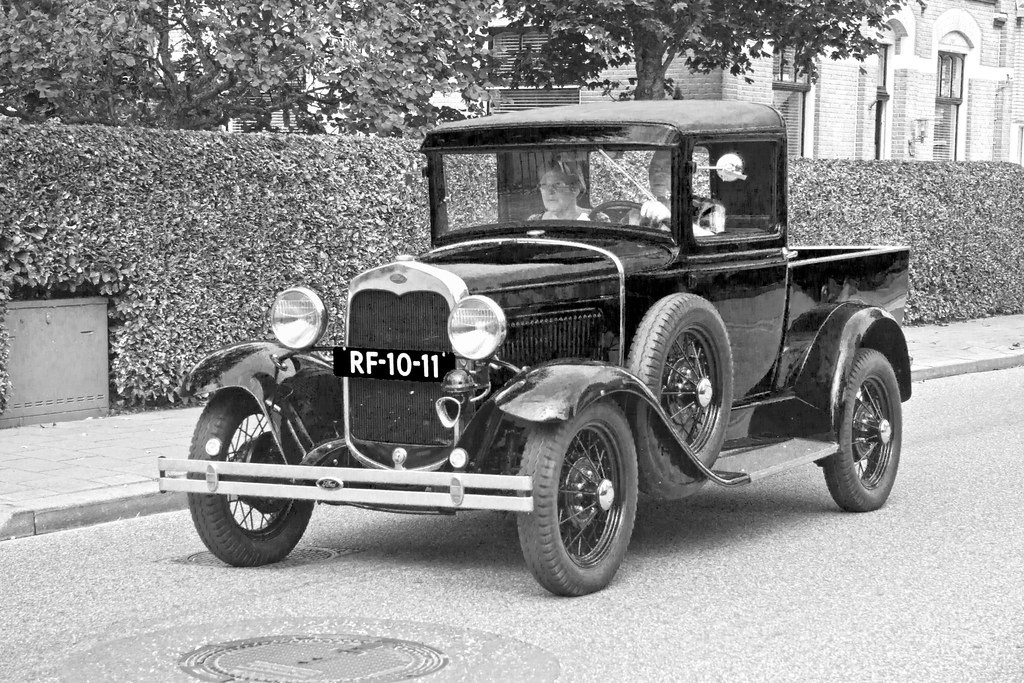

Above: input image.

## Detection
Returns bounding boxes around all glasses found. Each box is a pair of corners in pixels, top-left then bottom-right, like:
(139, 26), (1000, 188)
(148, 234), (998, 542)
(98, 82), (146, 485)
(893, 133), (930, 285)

(645, 162), (672, 174)
(535, 180), (573, 192)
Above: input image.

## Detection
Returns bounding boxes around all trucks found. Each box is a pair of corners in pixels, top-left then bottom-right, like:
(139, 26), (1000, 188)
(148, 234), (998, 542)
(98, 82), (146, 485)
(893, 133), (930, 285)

(157, 100), (913, 599)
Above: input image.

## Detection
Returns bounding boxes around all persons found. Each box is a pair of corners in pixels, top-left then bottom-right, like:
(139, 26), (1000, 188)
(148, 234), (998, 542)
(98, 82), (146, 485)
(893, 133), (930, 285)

(618, 149), (726, 238)
(525, 149), (610, 223)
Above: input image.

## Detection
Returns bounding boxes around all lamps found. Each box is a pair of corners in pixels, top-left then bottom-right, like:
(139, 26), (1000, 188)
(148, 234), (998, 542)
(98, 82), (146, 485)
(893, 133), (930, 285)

(907, 117), (929, 157)
